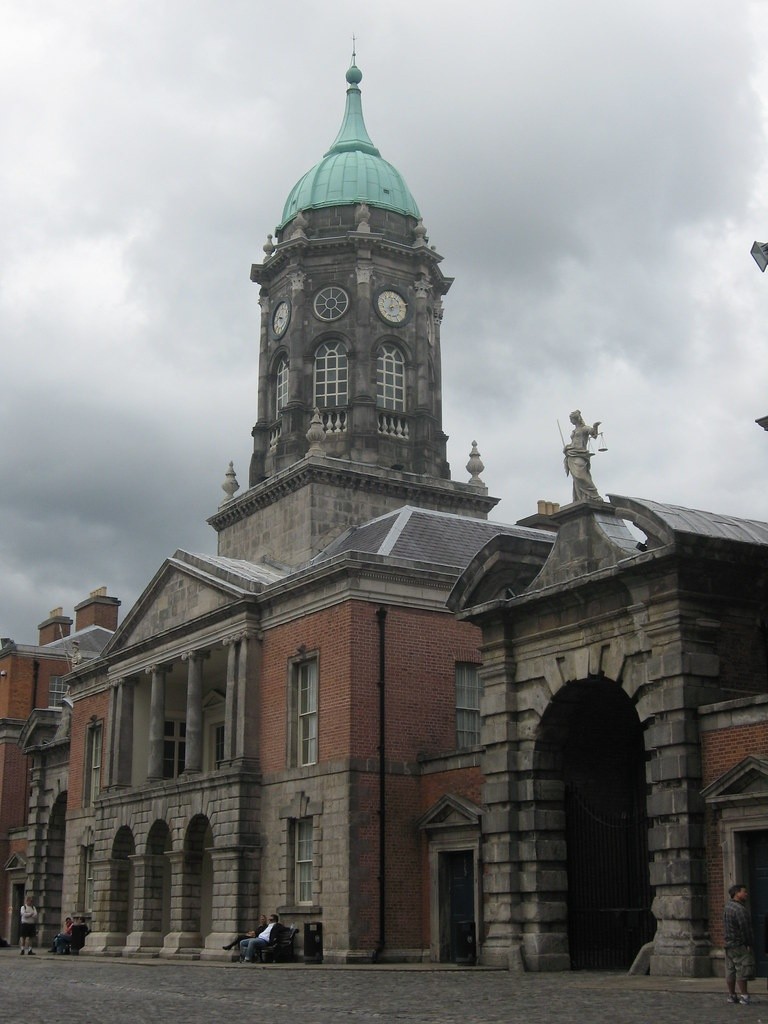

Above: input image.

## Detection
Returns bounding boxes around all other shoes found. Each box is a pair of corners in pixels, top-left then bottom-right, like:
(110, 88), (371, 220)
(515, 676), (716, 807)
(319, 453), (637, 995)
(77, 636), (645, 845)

(727, 994), (740, 1003)
(222, 945), (231, 951)
(739, 995), (761, 1005)
(28, 951), (35, 955)
(237, 959), (252, 964)
(20, 950), (25, 955)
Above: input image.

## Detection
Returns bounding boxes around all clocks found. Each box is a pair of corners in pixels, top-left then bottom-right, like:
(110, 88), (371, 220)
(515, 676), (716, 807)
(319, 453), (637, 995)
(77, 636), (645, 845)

(270, 298), (291, 341)
(372, 284), (412, 329)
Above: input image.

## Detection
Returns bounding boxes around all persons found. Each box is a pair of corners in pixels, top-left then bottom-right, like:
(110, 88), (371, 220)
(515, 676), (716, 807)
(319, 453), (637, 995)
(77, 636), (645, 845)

(222, 915), (268, 952)
(19, 896), (37, 955)
(562, 410), (604, 502)
(723, 885), (760, 1005)
(64, 640), (82, 696)
(239, 914), (300, 963)
(48, 917), (88, 954)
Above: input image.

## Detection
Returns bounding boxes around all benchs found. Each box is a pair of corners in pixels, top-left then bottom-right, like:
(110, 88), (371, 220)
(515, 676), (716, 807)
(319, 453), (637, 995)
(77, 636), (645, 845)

(231, 928), (300, 963)
(63, 930), (91, 954)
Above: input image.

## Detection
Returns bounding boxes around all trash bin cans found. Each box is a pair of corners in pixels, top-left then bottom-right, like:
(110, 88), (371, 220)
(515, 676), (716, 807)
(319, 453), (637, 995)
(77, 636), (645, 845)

(304, 921), (324, 965)
(457, 920), (476, 965)
